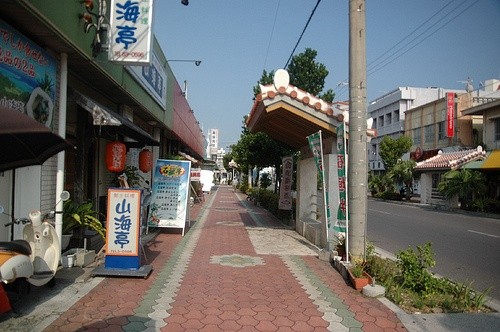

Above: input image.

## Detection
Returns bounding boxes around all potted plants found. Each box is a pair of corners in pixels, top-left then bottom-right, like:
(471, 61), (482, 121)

(329, 233), (368, 291)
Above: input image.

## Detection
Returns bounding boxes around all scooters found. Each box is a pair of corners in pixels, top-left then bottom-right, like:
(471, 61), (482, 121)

(0, 191), (72, 299)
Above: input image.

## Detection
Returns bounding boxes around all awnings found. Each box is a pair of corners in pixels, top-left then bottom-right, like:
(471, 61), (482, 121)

(459, 150), (500, 169)
(66, 83), (162, 150)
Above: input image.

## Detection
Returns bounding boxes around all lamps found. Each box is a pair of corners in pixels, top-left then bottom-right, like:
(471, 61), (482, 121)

(164, 57), (203, 72)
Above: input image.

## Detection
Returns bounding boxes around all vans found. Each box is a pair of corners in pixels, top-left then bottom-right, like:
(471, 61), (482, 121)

(399, 186), (413, 198)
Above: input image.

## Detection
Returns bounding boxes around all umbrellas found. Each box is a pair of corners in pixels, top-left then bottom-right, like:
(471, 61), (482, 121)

(1, 104), (72, 177)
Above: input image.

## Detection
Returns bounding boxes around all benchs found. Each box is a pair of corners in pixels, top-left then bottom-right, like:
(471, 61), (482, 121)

(305, 223), (322, 247)
(299, 217), (320, 236)
